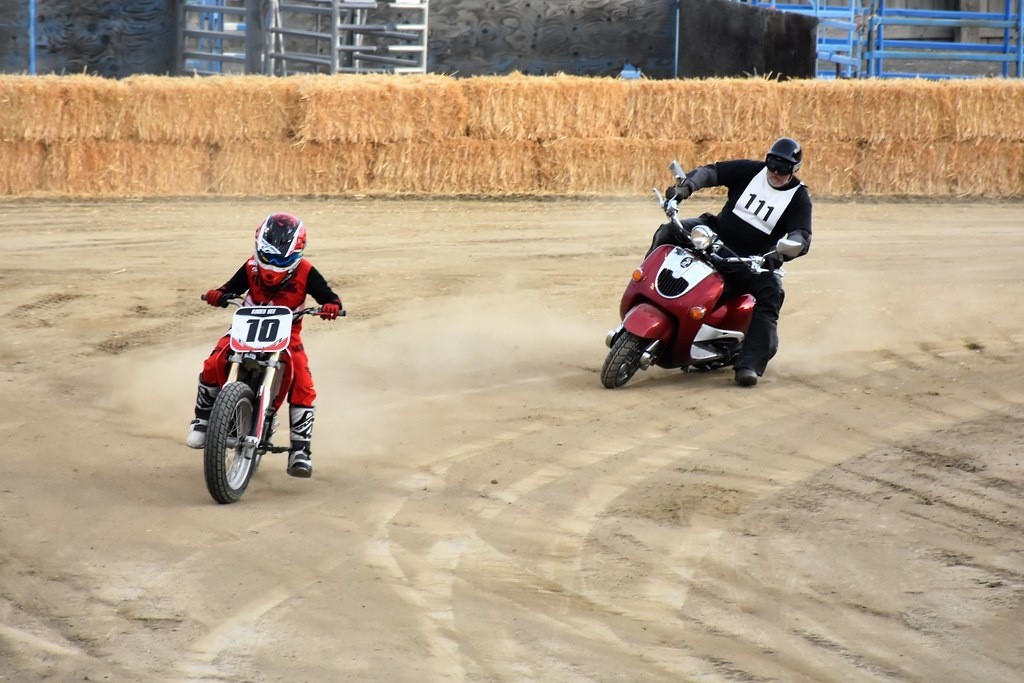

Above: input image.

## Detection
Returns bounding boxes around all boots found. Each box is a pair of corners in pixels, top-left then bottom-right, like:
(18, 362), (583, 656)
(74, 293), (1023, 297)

(287, 405), (315, 478)
(186, 373), (220, 449)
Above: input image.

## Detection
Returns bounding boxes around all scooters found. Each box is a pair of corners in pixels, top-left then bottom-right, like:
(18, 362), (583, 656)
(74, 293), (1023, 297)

(600, 157), (806, 390)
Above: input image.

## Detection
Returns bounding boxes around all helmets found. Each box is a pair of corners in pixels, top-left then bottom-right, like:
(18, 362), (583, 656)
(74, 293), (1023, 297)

(767, 138), (802, 165)
(254, 215), (306, 287)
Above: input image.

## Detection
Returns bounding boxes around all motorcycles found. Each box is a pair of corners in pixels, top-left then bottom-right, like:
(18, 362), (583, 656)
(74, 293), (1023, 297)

(201, 290), (346, 505)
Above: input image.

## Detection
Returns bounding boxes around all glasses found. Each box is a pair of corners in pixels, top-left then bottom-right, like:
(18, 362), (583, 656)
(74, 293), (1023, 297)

(767, 157), (802, 176)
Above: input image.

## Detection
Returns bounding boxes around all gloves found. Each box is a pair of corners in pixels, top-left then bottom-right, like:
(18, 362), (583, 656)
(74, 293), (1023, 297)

(206, 289), (227, 307)
(666, 184), (692, 203)
(320, 303), (339, 321)
(762, 246), (784, 276)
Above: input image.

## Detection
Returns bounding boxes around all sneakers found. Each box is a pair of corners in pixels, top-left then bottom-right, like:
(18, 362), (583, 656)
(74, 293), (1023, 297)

(735, 369), (757, 385)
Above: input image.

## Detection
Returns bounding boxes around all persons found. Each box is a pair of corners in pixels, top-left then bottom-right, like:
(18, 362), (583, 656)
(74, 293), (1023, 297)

(643, 138), (812, 386)
(185, 213), (342, 478)
